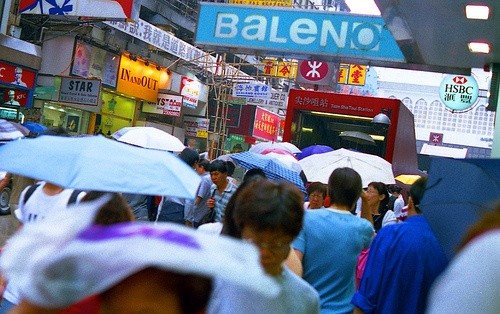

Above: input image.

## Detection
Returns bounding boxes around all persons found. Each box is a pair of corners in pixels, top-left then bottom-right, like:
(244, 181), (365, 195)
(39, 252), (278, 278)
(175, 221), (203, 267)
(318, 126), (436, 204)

(0, 149), (500, 314)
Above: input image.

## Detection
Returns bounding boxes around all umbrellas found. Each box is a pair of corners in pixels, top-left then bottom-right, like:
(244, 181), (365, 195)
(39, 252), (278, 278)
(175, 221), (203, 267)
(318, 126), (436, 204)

(338, 130), (377, 151)
(227, 138), (397, 194)
(0, 134), (201, 200)
(0, 118), (50, 139)
(394, 174), (421, 185)
(111, 127), (186, 151)
(418, 157), (500, 264)
